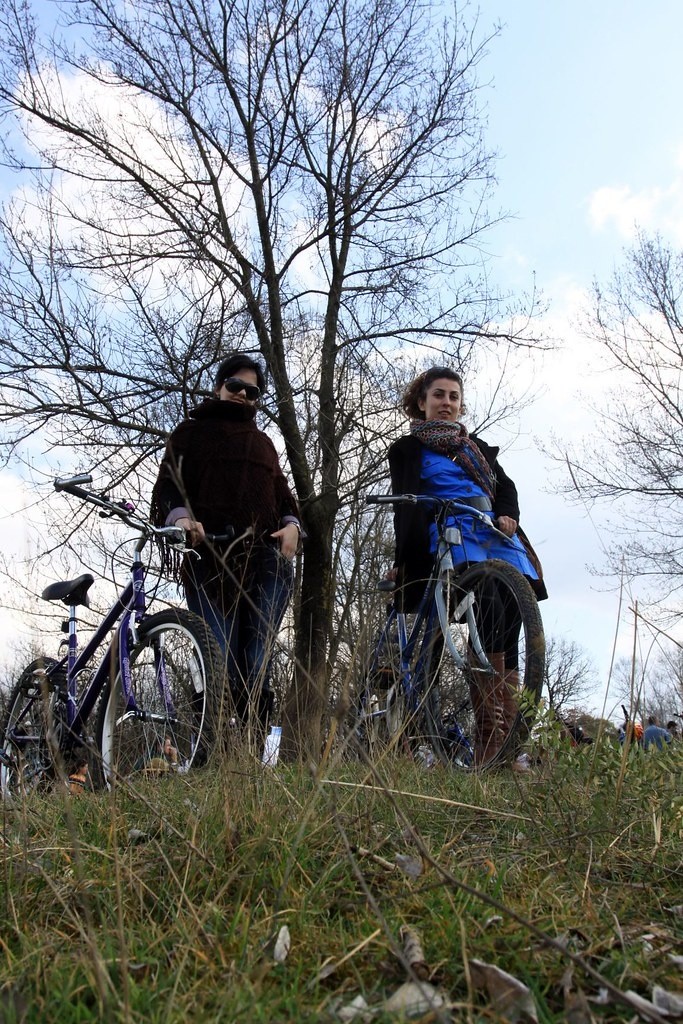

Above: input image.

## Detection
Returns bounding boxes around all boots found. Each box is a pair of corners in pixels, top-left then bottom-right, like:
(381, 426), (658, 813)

(235, 685), (275, 764)
(464, 649), (529, 777)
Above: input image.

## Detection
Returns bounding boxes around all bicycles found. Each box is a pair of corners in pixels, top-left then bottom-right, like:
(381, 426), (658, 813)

(359, 491), (546, 775)
(0, 474), (228, 801)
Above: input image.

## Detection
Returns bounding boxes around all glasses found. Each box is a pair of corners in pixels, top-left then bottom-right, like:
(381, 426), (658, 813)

(225, 377), (261, 401)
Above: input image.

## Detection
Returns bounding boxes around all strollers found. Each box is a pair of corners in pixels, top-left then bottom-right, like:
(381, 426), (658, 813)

(554, 711), (592, 746)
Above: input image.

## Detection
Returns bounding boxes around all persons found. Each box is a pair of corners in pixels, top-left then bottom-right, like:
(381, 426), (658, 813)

(133, 733), (178, 773)
(69, 759), (89, 793)
(618, 715), (678, 752)
(386, 367), (548, 775)
(149, 355), (309, 757)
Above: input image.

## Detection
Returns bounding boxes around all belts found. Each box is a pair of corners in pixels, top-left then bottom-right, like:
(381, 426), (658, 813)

(428, 496), (493, 523)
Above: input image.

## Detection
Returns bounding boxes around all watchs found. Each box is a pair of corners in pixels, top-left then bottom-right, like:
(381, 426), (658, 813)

(290, 522), (301, 531)
